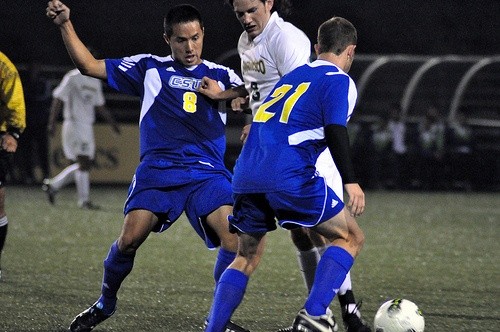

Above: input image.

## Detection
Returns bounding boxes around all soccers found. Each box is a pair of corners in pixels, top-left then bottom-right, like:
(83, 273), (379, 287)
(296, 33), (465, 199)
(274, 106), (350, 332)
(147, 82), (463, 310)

(373, 299), (425, 332)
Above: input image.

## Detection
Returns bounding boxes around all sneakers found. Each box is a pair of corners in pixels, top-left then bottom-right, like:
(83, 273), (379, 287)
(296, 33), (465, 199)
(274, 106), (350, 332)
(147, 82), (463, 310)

(204, 319), (250, 332)
(69, 297), (118, 331)
(292, 306), (345, 332)
(341, 301), (371, 332)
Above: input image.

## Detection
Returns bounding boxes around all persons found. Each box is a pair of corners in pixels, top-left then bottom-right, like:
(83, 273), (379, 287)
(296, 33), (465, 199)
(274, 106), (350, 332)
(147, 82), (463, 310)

(204, 16), (365, 332)
(46, 0), (252, 332)
(368, 101), (471, 192)
(0, 50), (27, 281)
(198, 0), (372, 332)
(41, 45), (121, 210)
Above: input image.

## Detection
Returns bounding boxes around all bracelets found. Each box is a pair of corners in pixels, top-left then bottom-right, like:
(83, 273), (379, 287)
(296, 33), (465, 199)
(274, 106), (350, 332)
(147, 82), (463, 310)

(9, 126), (20, 140)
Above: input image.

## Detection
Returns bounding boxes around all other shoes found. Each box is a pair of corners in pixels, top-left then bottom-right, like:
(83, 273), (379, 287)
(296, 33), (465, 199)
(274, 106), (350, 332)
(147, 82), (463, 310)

(78, 201), (99, 208)
(44, 179), (57, 204)
